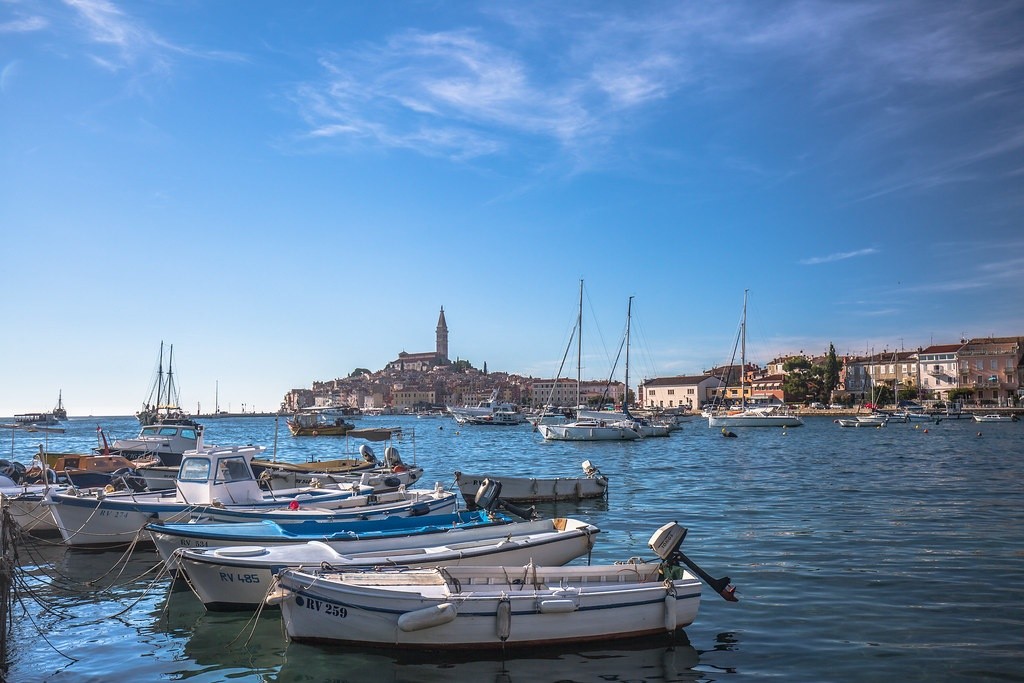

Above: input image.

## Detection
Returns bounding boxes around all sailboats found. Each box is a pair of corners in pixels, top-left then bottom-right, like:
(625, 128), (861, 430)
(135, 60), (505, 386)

(707, 288), (806, 428)
(133, 341), (202, 428)
(445, 277), (692, 442)
(833, 347), (973, 428)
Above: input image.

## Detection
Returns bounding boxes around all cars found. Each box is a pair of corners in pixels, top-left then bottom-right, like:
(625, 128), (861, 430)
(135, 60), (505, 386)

(730, 405), (742, 411)
(865, 400), (884, 410)
(829, 402), (846, 409)
(814, 402), (830, 410)
(897, 400), (916, 407)
(932, 399), (957, 409)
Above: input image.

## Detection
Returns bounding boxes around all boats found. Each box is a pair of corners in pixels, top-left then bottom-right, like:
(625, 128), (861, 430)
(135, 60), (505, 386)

(172, 514), (602, 615)
(972, 414), (1021, 423)
(1, 387), (459, 551)
(285, 404), (360, 436)
(140, 478), (544, 581)
(453, 459), (610, 507)
(261, 519), (742, 648)
